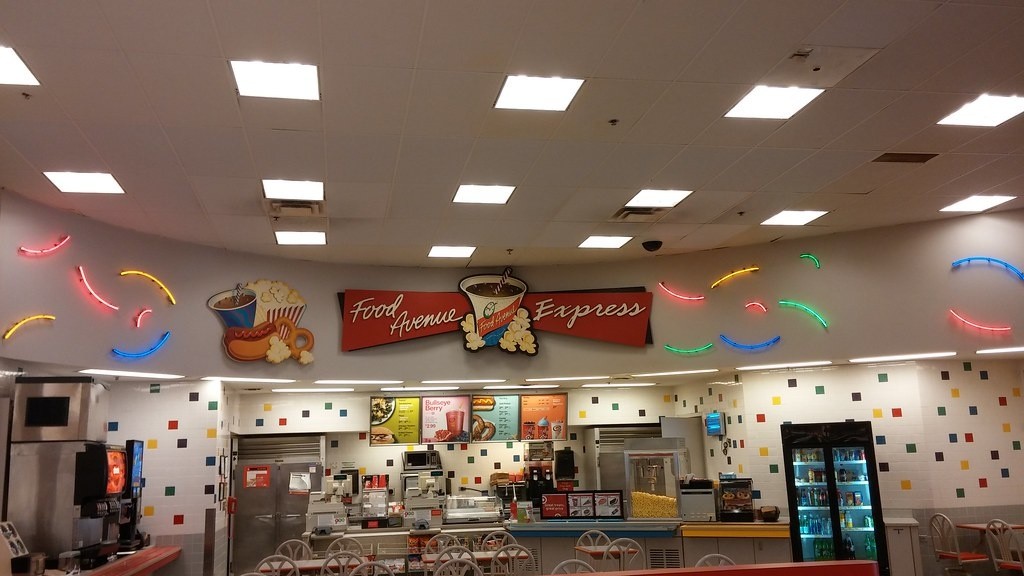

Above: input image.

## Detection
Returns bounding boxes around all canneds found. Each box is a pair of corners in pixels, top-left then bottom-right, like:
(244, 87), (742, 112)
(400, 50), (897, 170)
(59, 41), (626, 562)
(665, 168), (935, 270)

(832, 448), (866, 482)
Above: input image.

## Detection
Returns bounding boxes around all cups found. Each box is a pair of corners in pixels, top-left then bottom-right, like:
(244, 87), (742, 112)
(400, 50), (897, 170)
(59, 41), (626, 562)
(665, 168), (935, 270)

(551, 422), (564, 439)
(446, 411), (464, 436)
(517, 508), (525, 523)
(372, 476), (378, 488)
(523, 422), (535, 440)
(365, 481), (371, 488)
(572, 498), (578, 507)
(378, 474), (386, 488)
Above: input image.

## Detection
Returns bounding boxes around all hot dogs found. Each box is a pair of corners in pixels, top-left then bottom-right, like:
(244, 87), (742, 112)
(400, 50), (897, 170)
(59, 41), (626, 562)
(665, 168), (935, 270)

(472, 396), (495, 411)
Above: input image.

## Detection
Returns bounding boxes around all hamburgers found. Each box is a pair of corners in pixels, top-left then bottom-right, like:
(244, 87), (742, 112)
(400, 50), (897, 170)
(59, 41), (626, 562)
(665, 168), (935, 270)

(371, 427), (396, 444)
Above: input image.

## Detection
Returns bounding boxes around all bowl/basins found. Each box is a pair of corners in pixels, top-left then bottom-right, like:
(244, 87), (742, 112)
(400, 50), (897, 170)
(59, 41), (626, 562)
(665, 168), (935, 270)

(342, 462), (355, 467)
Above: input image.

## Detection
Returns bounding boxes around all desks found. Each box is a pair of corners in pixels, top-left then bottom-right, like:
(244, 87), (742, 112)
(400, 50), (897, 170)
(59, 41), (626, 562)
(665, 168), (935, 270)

(956, 523), (1024, 558)
(421, 550), (529, 576)
(72, 545), (183, 576)
(573, 545), (639, 572)
(260, 556), (367, 576)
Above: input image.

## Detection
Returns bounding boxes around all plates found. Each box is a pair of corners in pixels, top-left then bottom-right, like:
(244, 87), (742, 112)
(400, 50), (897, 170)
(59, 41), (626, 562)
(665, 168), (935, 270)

(371, 398), (396, 425)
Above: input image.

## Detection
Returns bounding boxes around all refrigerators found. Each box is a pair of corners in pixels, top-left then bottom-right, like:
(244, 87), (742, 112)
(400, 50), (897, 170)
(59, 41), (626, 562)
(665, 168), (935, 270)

(780, 417), (891, 576)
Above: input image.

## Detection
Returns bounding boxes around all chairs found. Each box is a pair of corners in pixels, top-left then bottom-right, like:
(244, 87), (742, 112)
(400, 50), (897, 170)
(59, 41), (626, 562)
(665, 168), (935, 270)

(421, 530), (536, 576)
(549, 529), (738, 576)
(984, 519), (1024, 576)
(239, 537), (397, 576)
(929, 513), (989, 576)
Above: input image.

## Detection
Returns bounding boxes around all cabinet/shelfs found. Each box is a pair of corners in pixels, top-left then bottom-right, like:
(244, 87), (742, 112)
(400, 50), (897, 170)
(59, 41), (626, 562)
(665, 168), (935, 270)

(882, 517), (923, 576)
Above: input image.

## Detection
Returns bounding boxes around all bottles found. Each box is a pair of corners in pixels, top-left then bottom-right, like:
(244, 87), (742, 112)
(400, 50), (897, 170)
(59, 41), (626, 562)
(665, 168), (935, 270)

(840, 510), (874, 528)
(537, 416), (549, 439)
(795, 467), (827, 482)
(796, 486), (829, 506)
(798, 513), (833, 535)
(834, 464), (868, 481)
(813, 538), (836, 561)
(525, 504), (533, 524)
(832, 447), (866, 461)
(792, 448), (824, 462)
(836, 487), (842, 505)
(843, 533), (877, 560)
